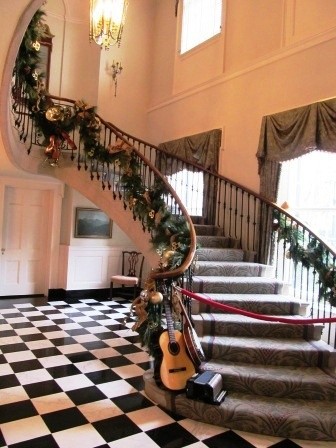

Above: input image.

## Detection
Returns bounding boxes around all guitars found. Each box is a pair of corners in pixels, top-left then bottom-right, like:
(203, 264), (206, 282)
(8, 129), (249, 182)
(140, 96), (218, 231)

(168, 277), (205, 367)
(153, 269), (196, 393)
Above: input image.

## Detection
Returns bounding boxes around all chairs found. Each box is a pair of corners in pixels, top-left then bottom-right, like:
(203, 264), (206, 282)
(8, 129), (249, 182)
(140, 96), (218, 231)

(110, 250), (145, 300)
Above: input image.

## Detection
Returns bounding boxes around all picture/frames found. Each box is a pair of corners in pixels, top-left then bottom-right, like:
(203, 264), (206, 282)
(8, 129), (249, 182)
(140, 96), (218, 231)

(75, 207), (113, 239)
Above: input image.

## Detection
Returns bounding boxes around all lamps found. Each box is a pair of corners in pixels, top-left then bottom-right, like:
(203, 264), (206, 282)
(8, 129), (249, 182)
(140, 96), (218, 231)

(89, 0), (129, 51)
